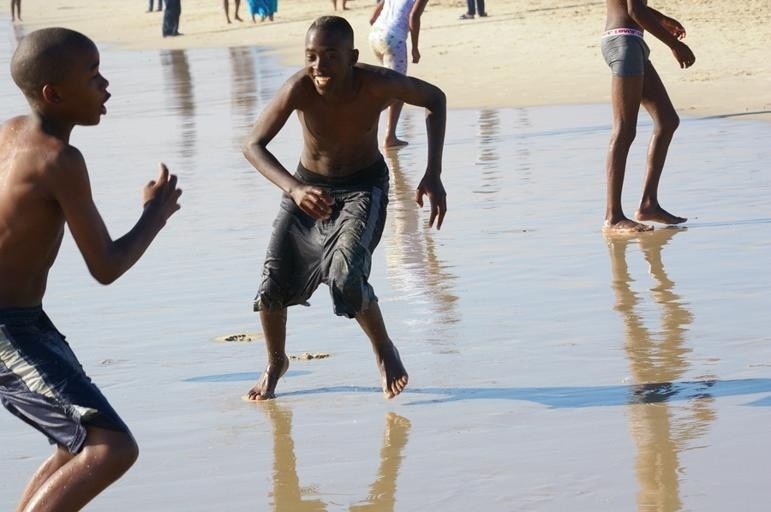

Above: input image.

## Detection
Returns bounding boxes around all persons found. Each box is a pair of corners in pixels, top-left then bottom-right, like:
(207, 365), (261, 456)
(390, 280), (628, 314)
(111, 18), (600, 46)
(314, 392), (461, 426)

(332, 0), (350, 10)
(162, 0), (183, 36)
(459, 0), (488, 19)
(367, 0), (430, 148)
(240, 14), (448, 401)
(0, 26), (184, 512)
(224, 0), (244, 24)
(11, 0), (23, 22)
(599, 0), (697, 232)
(247, 0), (278, 23)
(148, 0), (162, 11)
